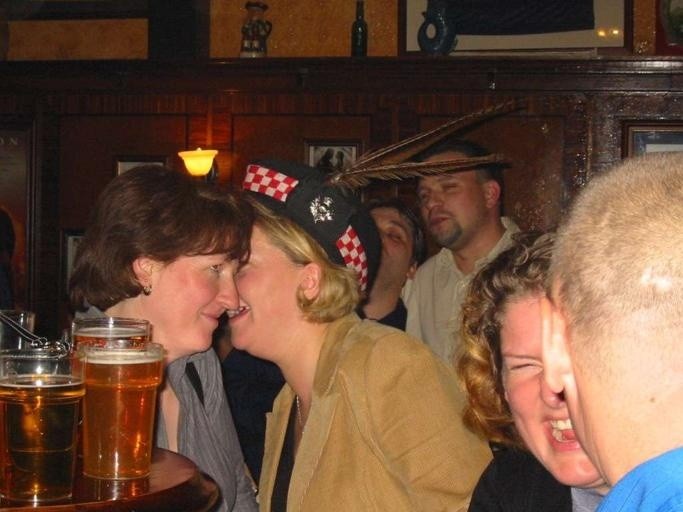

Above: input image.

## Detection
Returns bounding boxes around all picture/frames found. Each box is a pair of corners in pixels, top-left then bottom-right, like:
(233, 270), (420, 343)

(302, 139), (365, 207)
(111, 153), (168, 177)
(396, 0), (634, 57)
(58, 228), (92, 301)
(622, 118), (682, 160)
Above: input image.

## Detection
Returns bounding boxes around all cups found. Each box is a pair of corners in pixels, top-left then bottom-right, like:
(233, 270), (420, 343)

(0, 374), (83, 504)
(71, 316), (149, 365)
(78, 339), (163, 481)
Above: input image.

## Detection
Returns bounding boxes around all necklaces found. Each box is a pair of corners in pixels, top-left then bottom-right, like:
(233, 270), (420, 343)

(294, 395), (306, 435)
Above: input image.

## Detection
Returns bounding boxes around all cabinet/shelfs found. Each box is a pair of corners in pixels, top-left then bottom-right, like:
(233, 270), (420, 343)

(210, 89), (396, 202)
(592, 89), (683, 173)
(402, 89), (590, 256)
(28, 90), (207, 331)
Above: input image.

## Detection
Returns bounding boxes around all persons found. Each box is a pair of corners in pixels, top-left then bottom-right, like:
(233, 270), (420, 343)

(65, 164), (260, 512)
(356, 195), (427, 330)
(538, 151), (683, 511)
(224, 158), (495, 512)
(456, 227), (611, 512)
(400, 139), (524, 361)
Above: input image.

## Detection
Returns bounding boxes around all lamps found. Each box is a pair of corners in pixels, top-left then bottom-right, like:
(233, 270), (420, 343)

(175, 148), (218, 178)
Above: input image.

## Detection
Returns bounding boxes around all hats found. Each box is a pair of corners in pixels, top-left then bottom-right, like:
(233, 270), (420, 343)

(242, 91), (525, 308)
